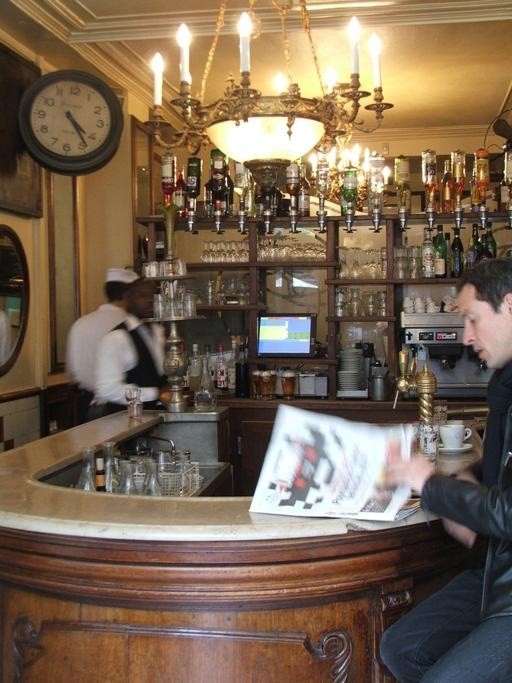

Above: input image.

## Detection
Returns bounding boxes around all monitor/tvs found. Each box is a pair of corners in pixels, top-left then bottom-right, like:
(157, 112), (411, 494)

(257, 313), (317, 357)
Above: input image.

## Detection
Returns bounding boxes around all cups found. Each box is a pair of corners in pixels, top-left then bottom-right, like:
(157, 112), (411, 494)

(201, 242), (326, 261)
(142, 259), (197, 319)
(403, 296), (439, 312)
(334, 246), (421, 279)
(439, 426), (472, 447)
(335, 287), (387, 316)
(250, 372), (295, 399)
(204, 276), (251, 305)
(122, 385), (142, 419)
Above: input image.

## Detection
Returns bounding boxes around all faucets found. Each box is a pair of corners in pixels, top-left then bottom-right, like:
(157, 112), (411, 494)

(134, 434), (175, 455)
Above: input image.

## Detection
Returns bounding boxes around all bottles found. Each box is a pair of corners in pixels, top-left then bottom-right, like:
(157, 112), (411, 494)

(185, 336), (244, 396)
(76, 442), (161, 498)
(422, 225), (497, 278)
(152, 151), (511, 236)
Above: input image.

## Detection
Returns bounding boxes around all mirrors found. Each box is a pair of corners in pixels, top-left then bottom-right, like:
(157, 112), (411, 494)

(0, 223), (30, 376)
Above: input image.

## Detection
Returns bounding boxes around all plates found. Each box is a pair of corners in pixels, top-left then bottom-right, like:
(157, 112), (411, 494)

(435, 443), (474, 452)
(336, 348), (365, 391)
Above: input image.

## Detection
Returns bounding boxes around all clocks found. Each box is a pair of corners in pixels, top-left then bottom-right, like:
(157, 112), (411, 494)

(16, 68), (125, 176)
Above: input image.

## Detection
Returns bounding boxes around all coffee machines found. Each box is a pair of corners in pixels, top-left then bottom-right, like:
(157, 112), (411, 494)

(400, 313), (497, 399)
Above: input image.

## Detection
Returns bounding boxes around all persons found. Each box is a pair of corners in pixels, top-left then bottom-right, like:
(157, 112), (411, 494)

(64, 266), (139, 428)
(380, 257), (511, 683)
(83, 279), (170, 424)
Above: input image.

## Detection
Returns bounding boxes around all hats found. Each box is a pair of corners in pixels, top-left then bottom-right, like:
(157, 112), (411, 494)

(106, 269), (139, 283)
